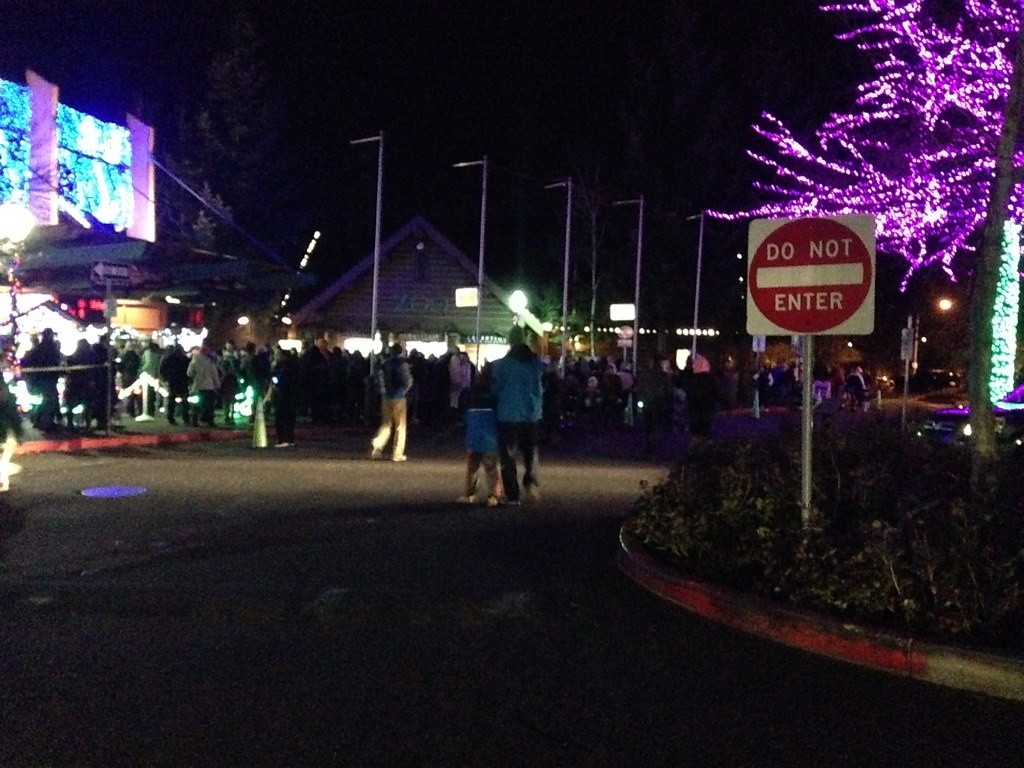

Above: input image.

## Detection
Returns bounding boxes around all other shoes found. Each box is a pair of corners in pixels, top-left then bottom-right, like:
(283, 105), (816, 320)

(465, 496), (474, 504)
(274, 442), (289, 447)
(501, 500), (520, 506)
(487, 497), (498, 507)
(522, 472), (538, 489)
(393, 455), (407, 462)
(372, 450), (381, 459)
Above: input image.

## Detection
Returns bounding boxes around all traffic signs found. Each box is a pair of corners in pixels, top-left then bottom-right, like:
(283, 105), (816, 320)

(92, 260), (129, 282)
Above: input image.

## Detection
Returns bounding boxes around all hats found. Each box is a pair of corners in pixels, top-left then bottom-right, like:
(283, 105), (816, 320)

(506, 327), (523, 344)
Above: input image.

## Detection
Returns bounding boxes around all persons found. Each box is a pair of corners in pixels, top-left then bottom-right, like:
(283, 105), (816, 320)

(0, 324), (877, 509)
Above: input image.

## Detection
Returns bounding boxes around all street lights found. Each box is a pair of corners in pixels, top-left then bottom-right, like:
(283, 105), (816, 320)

(687, 213), (705, 365)
(349, 130), (384, 376)
(544, 177), (573, 378)
(611, 196), (644, 377)
(454, 155), (488, 374)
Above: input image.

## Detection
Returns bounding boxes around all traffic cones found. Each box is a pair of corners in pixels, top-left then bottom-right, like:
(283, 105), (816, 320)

(248, 395), (270, 449)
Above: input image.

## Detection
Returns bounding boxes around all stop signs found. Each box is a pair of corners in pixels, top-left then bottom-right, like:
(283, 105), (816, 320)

(746, 212), (877, 336)
